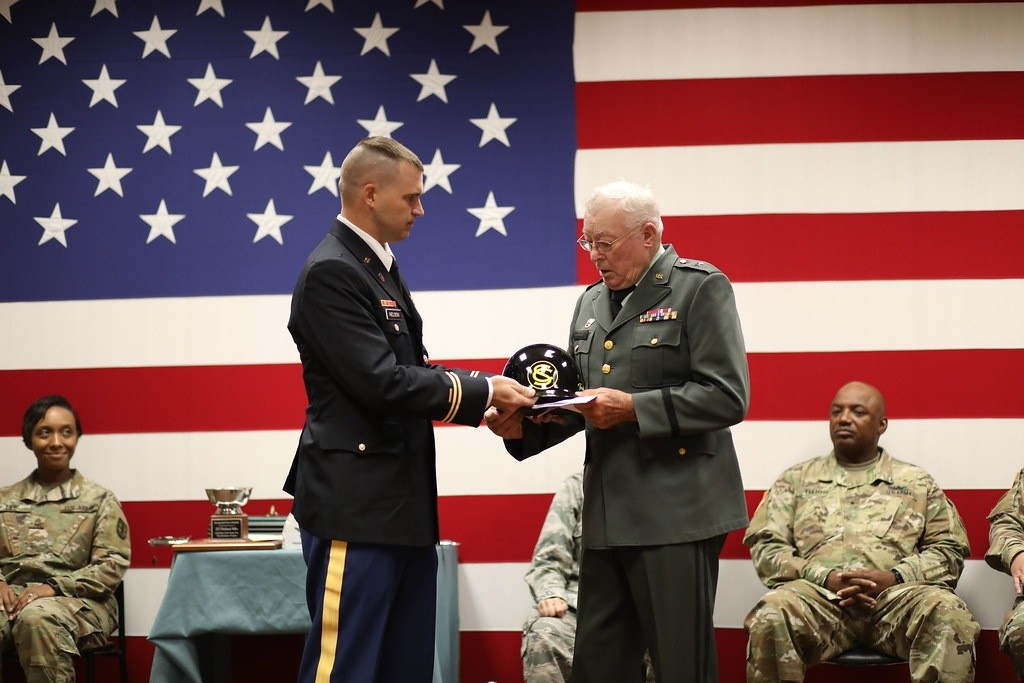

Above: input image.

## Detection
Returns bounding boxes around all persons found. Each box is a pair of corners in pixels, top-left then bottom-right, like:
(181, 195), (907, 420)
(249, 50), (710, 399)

(0, 393), (131, 683)
(988, 467), (1024, 683)
(283, 137), (539, 683)
(482, 181), (752, 683)
(522, 464), (656, 683)
(742, 380), (981, 683)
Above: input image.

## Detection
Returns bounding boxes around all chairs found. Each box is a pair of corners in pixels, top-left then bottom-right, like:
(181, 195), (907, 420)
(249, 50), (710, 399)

(826, 647), (904, 665)
(76, 579), (125, 683)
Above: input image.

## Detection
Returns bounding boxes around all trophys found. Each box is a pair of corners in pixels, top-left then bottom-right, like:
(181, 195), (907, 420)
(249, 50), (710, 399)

(204, 487), (253, 543)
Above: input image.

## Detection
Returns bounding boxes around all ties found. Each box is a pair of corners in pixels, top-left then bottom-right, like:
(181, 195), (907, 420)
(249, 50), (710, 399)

(609, 284), (637, 321)
(391, 255), (403, 299)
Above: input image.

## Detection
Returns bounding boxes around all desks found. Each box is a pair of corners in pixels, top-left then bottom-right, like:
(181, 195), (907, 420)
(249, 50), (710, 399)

(146, 541), (459, 683)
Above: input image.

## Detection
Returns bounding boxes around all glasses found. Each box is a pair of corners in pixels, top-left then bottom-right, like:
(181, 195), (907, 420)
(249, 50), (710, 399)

(576, 223), (643, 252)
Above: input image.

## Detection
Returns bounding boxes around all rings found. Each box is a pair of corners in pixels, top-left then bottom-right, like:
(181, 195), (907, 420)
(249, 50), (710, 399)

(28, 594), (32, 598)
